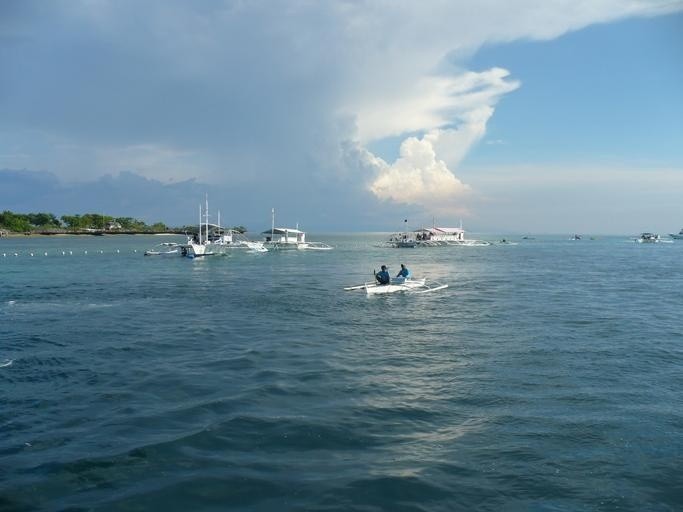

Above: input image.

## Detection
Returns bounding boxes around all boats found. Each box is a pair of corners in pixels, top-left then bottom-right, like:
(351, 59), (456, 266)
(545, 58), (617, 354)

(143, 193), (335, 259)
(383, 224), (536, 248)
(364, 274), (427, 297)
(568, 234), (582, 241)
(630, 229), (682, 245)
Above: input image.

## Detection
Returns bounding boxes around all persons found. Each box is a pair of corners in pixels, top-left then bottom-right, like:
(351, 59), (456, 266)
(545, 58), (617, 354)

(396, 263), (411, 280)
(376, 265), (390, 285)
